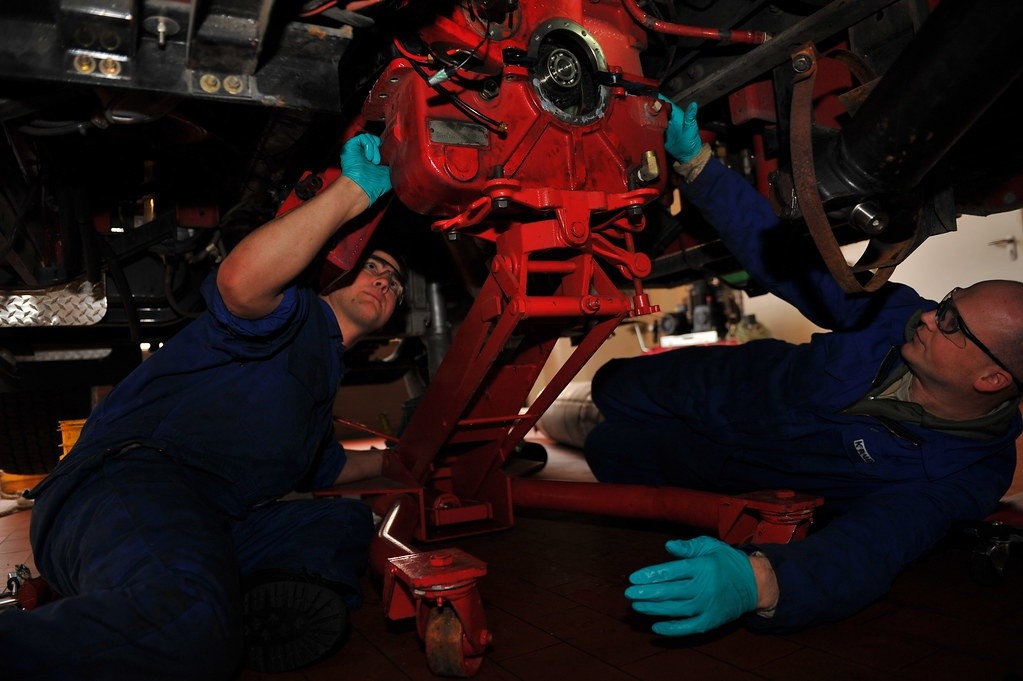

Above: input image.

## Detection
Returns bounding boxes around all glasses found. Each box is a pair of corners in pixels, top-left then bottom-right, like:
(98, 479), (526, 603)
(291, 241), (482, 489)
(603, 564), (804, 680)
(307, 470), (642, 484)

(357, 252), (407, 305)
(935, 286), (1015, 382)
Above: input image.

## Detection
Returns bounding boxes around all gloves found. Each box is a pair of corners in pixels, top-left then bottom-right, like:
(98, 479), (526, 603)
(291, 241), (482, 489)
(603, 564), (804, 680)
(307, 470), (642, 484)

(657, 94), (702, 165)
(340, 132), (392, 209)
(625, 534), (758, 635)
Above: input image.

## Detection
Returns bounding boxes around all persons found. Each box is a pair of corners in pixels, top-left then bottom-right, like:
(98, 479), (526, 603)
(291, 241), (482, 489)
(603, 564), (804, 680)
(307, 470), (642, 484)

(0, 134), (404, 681)
(533, 93), (1023, 637)
